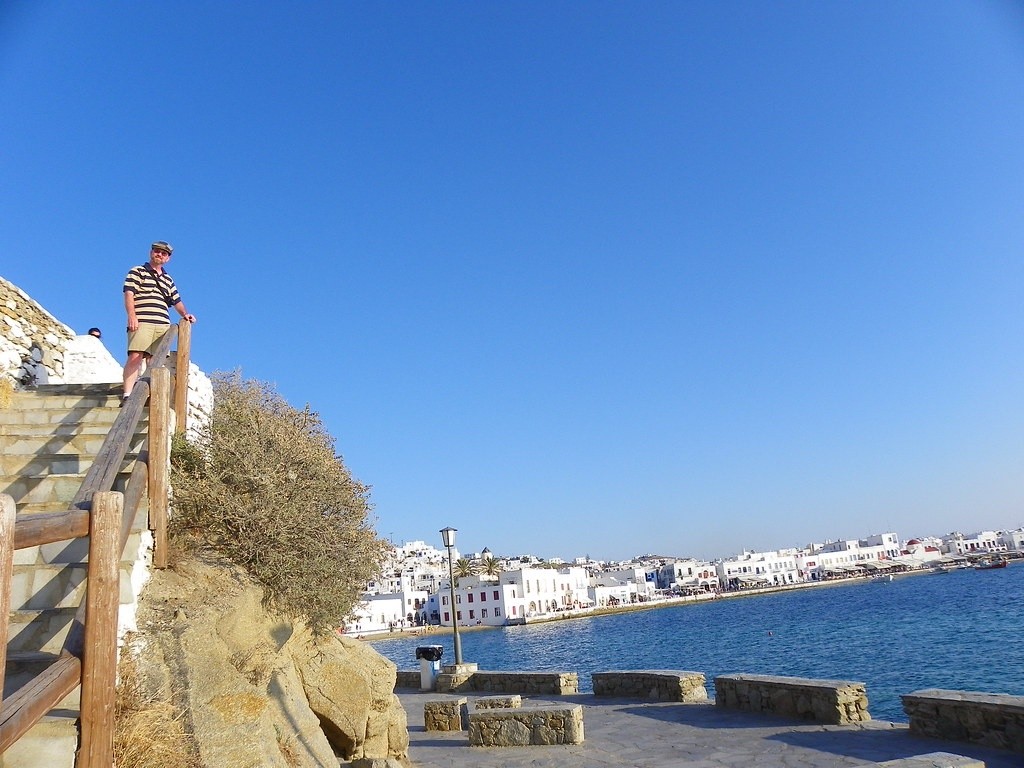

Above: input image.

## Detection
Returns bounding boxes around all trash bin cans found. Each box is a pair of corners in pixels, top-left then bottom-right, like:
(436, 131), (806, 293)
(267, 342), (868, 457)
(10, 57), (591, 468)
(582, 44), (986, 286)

(416, 644), (443, 690)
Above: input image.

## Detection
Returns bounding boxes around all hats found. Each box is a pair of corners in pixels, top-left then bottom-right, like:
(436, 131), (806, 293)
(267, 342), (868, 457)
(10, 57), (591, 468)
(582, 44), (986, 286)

(152, 240), (173, 254)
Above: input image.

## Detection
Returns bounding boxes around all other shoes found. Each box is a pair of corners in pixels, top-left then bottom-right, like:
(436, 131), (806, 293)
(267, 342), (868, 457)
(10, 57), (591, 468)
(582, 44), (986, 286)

(119, 396), (130, 407)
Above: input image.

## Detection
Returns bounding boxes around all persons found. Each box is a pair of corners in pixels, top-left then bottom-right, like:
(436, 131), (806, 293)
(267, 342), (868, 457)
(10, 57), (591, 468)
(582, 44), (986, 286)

(665, 584), (736, 599)
(389, 619), (432, 634)
(476, 619), (482, 626)
(120, 241), (197, 409)
(88, 328), (101, 339)
(356, 625), (362, 630)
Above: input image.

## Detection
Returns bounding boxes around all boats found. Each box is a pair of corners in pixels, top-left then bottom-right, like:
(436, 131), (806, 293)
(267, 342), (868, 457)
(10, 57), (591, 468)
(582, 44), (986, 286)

(974, 556), (1007, 570)
(871, 572), (894, 582)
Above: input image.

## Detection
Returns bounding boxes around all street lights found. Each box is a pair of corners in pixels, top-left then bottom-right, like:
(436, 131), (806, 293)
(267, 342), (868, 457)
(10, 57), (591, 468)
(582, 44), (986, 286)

(440, 525), (463, 664)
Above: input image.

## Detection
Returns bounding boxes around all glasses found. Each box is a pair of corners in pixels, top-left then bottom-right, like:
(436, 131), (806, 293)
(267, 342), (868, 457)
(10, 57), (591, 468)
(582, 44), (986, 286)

(90, 334), (101, 338)
(153, 248), (168, 257)
